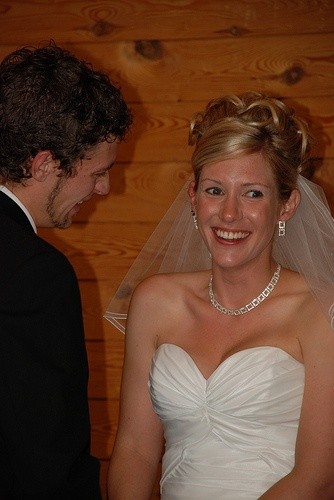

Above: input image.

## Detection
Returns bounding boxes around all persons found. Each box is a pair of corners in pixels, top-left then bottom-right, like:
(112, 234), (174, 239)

(103, 88), (334, 500)
(1, 35), (132, 498)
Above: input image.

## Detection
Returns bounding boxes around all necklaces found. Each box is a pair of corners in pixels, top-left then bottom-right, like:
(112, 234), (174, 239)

(208, 265), (284, 316)
